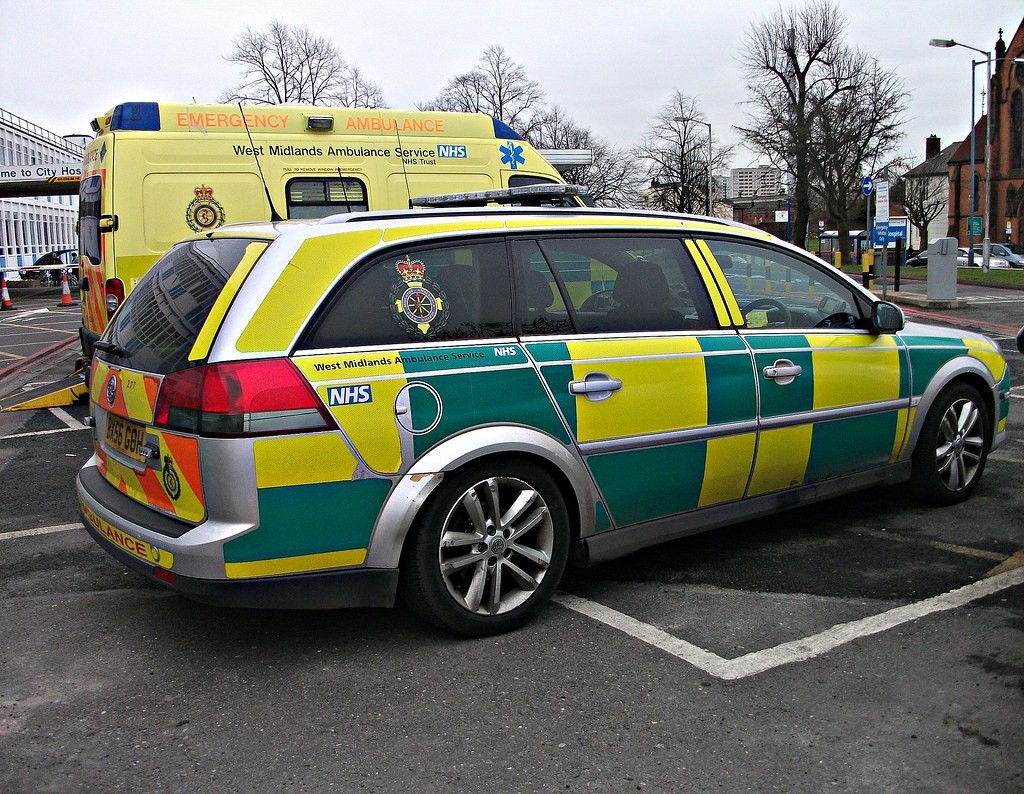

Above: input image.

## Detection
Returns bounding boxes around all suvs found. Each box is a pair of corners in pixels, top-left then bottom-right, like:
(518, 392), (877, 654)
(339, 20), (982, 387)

(973, 241), (1024, 270)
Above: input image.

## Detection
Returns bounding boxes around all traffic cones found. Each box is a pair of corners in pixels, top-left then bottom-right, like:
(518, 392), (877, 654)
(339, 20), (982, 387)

(0, 278), (17, 311)
(56, 274), (79, 307)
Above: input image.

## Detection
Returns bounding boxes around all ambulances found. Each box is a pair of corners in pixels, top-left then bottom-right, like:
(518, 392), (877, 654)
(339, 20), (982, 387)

(0, 100), (630, 414)
(73, 182), (1012, 640)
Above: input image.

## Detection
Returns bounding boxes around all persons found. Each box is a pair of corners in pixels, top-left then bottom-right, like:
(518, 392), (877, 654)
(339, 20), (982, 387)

(71, 253), (79, 280)
(45, 253), (63, 287)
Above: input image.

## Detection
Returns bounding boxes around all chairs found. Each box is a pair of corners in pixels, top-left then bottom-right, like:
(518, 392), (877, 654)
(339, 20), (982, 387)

(322, 263), (689, 341)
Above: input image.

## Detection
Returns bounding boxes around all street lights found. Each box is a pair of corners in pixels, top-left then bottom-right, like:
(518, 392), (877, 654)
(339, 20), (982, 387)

(930, 38), (993, 269)
(968, 58), (1024, 267)
(672, 115), (713, 217)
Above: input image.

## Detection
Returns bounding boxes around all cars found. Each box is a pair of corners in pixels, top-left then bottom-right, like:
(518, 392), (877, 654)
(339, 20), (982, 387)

(957, 246), (1011, 271)
(905, 249), (927, 268)
(18, 247), (79, 286)
